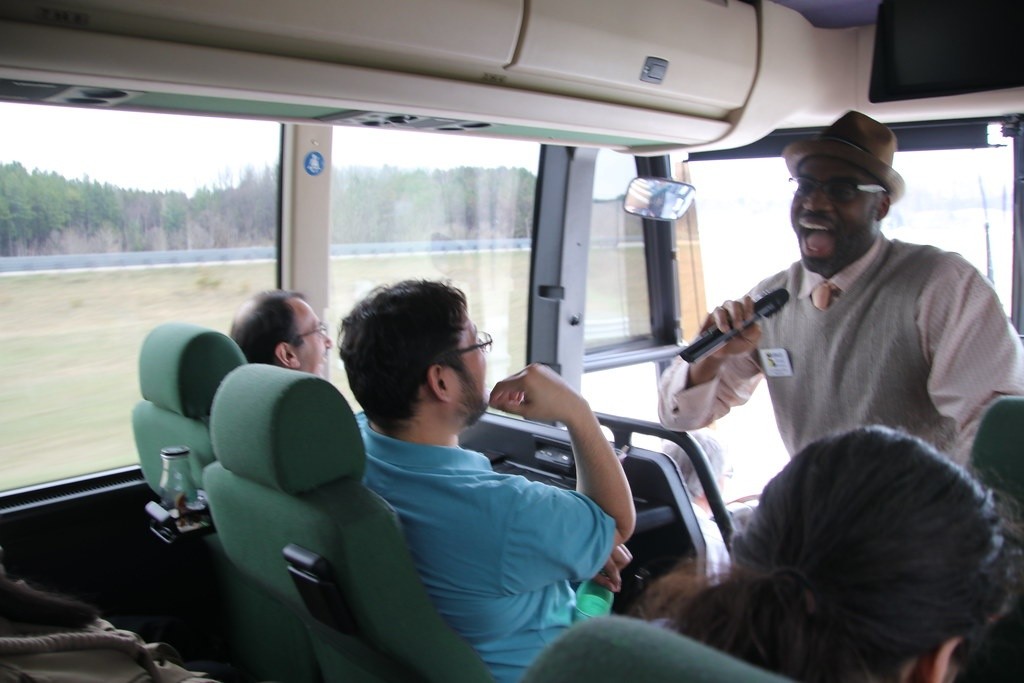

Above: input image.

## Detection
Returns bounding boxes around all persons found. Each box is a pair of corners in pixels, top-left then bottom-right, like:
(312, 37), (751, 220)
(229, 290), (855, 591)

(656, 110), (1024, 472)
(229, 288), (333, 378)
(665, 422), (1020, 683)
(338, 278), (637, 683)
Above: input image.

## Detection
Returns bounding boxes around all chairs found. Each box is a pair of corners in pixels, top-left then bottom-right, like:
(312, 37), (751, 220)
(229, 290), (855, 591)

(134, 328), (313, 682)
(200, 361), (497, 682)
(967, 396), (1024, 525)
(518, 614), (798, 683)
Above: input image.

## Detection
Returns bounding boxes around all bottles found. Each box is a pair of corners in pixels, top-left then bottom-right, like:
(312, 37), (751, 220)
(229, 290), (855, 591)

(573, 569), (614, 623)
(159, 447), (196, 510)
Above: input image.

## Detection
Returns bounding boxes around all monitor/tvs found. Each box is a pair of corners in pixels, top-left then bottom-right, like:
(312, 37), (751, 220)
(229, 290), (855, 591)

(869, 0), (1024, 104)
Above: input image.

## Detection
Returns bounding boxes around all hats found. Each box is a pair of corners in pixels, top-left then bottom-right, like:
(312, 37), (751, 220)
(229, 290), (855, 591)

(784, 110), (905, 204)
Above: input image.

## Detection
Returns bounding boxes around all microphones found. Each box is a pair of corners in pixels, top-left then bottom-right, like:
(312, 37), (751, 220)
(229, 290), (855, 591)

(680, 289), (789, 365)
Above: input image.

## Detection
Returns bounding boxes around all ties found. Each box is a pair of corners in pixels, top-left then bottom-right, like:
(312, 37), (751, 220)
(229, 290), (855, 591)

(811, 283), (830, 310)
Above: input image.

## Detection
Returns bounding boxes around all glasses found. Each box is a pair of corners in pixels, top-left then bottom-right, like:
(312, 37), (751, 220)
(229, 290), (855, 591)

(789, 175), (887, 204)
(424, 331), (494, 385)
(285, 321), (328, 348)
